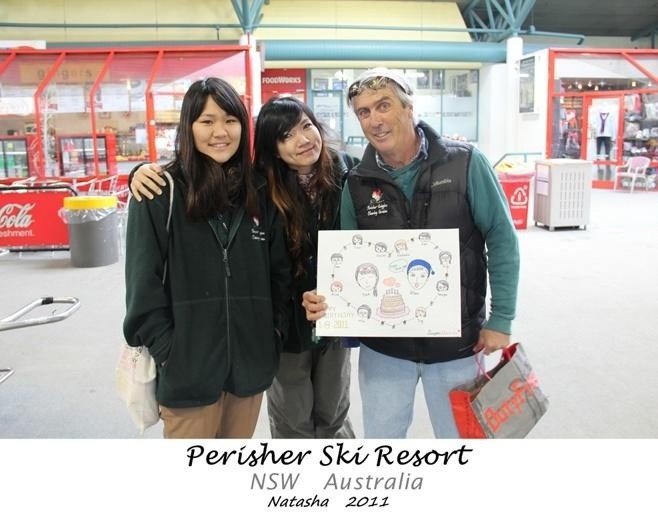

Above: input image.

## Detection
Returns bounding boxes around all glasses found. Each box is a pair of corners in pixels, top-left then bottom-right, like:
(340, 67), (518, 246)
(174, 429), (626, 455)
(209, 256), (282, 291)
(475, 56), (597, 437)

(348, 76), (406, 100)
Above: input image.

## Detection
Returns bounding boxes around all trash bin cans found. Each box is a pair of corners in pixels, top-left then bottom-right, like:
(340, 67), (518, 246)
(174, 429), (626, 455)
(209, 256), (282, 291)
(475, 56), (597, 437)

(63, 196), (118, 267)
(497, 162), (535, 229)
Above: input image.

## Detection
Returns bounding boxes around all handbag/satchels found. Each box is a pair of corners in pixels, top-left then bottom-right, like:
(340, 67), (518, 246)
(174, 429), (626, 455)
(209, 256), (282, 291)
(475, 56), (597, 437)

(126, 348), (158, 431)
(449, 343), (549, 439)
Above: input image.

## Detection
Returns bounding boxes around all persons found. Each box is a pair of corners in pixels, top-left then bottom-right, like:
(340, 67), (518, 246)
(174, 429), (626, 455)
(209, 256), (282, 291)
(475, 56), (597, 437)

(594, 108), (615, 171)
(122, 76), (292, 438)
(301, 66), (523, 439)
(128, 98), (360, 438)
(326, 232), (454, 326)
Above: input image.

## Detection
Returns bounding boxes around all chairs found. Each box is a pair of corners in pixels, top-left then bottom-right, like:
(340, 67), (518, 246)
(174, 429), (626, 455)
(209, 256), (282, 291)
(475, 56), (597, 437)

(0, 175), (131, 235)
(613, 155), (650, 194)
